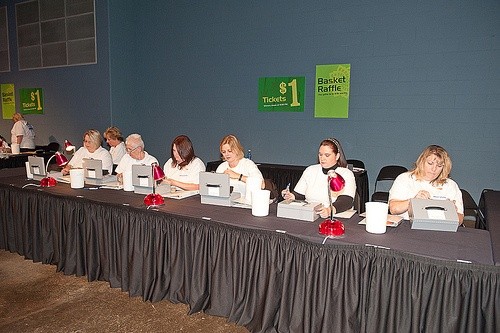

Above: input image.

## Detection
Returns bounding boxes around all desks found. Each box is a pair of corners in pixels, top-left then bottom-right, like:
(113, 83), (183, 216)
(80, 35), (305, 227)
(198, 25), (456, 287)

(0, 152), (500, 333)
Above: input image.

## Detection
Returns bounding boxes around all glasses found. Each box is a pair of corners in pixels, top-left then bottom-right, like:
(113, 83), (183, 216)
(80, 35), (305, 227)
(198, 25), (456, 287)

(127, 147), (139, 154)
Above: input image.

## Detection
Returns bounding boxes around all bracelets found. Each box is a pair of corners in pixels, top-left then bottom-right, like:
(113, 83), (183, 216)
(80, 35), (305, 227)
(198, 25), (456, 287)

(239, 174), (243, 181)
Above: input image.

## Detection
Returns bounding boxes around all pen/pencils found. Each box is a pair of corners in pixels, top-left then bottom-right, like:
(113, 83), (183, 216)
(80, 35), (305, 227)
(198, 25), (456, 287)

(83, 187), (99, 189)
(282, 183), (291, 198)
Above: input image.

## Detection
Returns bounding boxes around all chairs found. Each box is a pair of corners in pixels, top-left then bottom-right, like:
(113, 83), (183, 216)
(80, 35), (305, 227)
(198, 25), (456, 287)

(38, 139), (489, 226)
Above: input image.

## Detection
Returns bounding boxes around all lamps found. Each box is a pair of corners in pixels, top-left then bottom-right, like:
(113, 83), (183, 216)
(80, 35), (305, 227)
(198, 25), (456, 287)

(61, 139), (73, 154)
(40, 151), (69, 187)
(144, 162), (165, 208)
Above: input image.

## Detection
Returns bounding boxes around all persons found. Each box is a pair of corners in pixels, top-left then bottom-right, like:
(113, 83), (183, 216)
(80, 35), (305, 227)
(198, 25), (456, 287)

(11, 113), (35, 153)
(114, 134), (159, 182)
(281, 138), (357, 219)
(0, 135), (10, 148)
(103, 127), (127, 175)
(163, 135), (206, 190)
(388, 145), (464, 226)
(61, 130), (113, 175)
(215, 135), (265, 192)
